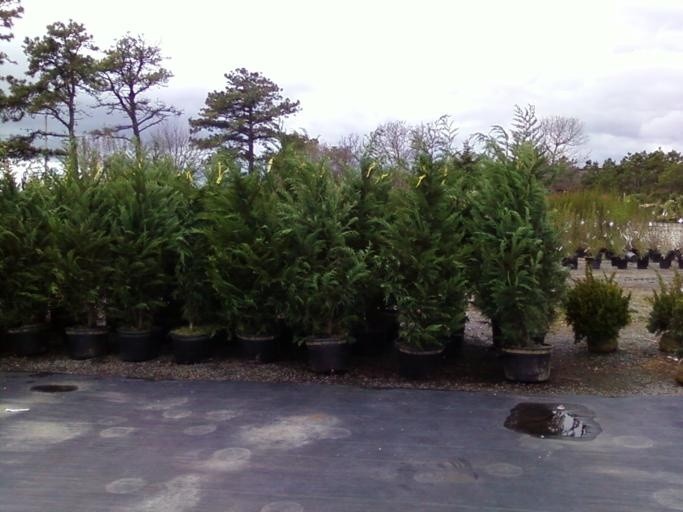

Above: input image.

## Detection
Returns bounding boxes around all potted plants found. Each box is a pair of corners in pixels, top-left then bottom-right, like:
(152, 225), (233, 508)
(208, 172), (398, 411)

(546, 189), (683, 268)
(645, 267), (682, 356)
(1, 127), (569, 383)
(563, 269), (635, 354)
